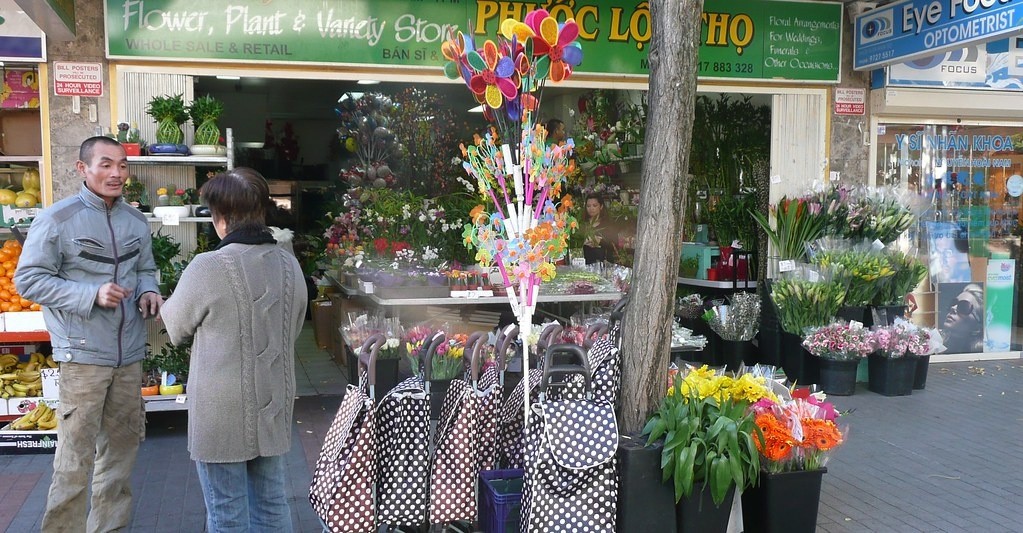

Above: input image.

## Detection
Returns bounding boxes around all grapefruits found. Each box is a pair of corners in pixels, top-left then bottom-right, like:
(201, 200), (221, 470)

(0, 186), (41, 208)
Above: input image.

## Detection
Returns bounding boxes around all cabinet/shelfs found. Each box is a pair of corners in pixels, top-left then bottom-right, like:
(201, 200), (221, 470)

(267, 181), (339, 274)
(125, 128), (235, 412)
(601, 149), (758, 288)
(0, 62), (60, 456)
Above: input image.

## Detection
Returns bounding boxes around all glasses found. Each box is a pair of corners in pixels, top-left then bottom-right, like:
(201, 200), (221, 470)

(932, 247), (955, 257)
(951, 297), (978, 321)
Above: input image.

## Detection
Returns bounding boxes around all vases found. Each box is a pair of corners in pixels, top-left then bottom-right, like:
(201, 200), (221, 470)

(870, 305), (907, 327)
(344, 345), (401, 403)
(603, 196), (620, 209)
(425, 377), (458, 421)
(620, 142), (637, 157)
(913, 353), (930, 391)
(835, 304), (870, 327)
(767, 255), (809, 279)
(675, 465), (736, 533)
(529, 352), (580, 396)
(739, 467), (828, 533)
(816, 358), (862, 395)
(452, 285), (477, 291)
(607, 144), (620, 159)
(482, 284), (491, 290)
(780, 331), (818, 385)
(500, 373), (523, 406)
(344, 272), (360, 288)
(867, 355), (918, 398)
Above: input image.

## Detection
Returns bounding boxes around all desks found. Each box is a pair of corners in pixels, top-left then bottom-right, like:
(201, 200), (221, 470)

(315, 261), (333, 270)
(324, 270), (334, 283)
(364, 285), (623, 331)
(331, 274), (358, 295)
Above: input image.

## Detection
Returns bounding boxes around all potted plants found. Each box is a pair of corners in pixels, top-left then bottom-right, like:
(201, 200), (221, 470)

(635, 96), (646, 156)
(141, 373), (159, 396)
(677, 292), (706, 361)
(186, 187), (202, 216)
(153, 184), (189, 218)
(123, 176), (151, 213)
(106, 122), (143, 155)
(147, 91), (189, 156)
(148, 228), (183, 299)
(567, 210), (606, 266)
(159, 372), (183, 395)
(188, 96), (228, 157)
(694, 170), (712, 243)
(730, 98), (760, 280)
(705, 290), (763, 371)
(698, 109), (735, 281)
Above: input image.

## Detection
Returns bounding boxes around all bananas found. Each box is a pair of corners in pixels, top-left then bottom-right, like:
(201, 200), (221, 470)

(0, 352), (61, 430)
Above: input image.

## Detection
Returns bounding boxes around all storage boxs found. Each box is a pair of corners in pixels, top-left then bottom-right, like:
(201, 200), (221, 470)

(467, 266), (504, 283)
(681, 242), (719, 280)
(331, 295), (366, 343)
(359, 277), (374, 294)
(373, 284), (449, 299)
(8, 398), (44, 421)
(479, 468), (524, 533)
(334, 340), (347, 365)
(5, 311), (48, 332)
(309, 299), (332, 350)
(432, 319), (495, 337)
(0, 203), (42, 227)
(0, 418), (58, 456)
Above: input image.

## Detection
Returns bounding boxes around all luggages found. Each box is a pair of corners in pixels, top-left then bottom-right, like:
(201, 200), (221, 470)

(309, 298), (629, 533)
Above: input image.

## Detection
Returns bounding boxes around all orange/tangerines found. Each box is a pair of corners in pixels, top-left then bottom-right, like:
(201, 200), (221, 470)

(0, 240), (42, 313)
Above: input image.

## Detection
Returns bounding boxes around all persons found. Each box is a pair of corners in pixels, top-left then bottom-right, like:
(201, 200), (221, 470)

(940, 284), (983, 354)
(574, 193), (619, 265)
(157, 164), (308, 532)
(931, 223), (970, 285)
(13, 136), (165, 533)
(545, 119), (566, 151)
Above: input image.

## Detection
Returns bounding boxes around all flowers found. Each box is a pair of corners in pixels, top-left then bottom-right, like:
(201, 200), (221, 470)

(446, 269), (480, 285)
(476, 335), (524, 369)
(600, 122), (620, 143)
(341, 312), (404, 358)
(803, 322), (875, 360)
(861, 187), (935, 252)
(643, 367), (779, 509)
(827, 250), (894, 305)
(909, 325), (948, 355)
(876, 247), (929, 305)
(322, 187), (464, 260)
(616, 109), (640, 141)
(600, 184), (622, 196)
(343, 256), (367, 273)
(403, 321), (468, 380)
(810, 185), (871, 251)
(481, 272), (491, 285)
(528, 321), (584, 355)
(746, 191), (829, 260)
(770, 278), (848, 334)
(735, 386), (858, 475)
(868, 321), (920, 358)
